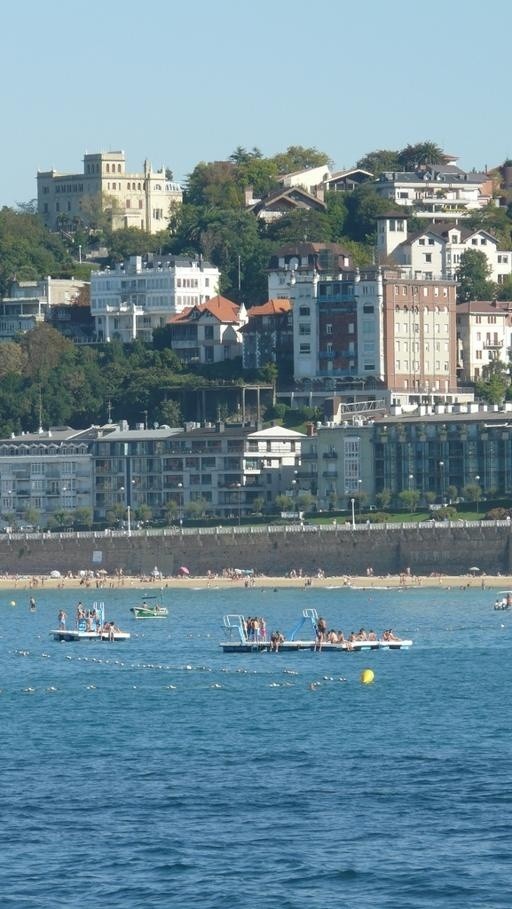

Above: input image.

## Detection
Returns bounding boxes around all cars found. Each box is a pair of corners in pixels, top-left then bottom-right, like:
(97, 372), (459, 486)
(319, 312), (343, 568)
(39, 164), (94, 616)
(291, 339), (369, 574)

(1, 527), (14, 533)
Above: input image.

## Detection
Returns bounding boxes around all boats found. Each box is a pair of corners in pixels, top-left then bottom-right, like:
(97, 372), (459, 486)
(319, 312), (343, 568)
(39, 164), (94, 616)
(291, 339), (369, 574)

(493, 590), (512, 610)
(218, 608), (412, 652)
(50, 601), (131, 642)
(129, 595), (171, 619)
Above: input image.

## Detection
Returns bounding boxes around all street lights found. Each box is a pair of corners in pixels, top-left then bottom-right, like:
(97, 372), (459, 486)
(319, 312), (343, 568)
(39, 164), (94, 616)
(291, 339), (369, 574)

(237, 483), (241, 526)
(439, 462), (445, 503)
(410, 476), (415, 513)
(358, 479), (362, 510)
(350, 499), (356, 529)
(9, 490), (13, 512)
(120, 487), (124, 503)
(62, 487), (66, 510)
(293, 470), (298, 509)
(474, 474), (481, 513)
(291, 480), (296, 522)
(178, 482), (184, 532)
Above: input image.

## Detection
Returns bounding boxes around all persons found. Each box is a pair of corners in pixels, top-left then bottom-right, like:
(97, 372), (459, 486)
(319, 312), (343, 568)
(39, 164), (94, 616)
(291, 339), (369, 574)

(30, 597), (36, 608)
(209, 568), (512, 610)
(314, 618), (398, 652)
(74, 601), (119, 643)
(58, 610), (67, 629)
(6, 567), (195, 591)
(143, 602), (160, 610)
(244, 616), (285, 652)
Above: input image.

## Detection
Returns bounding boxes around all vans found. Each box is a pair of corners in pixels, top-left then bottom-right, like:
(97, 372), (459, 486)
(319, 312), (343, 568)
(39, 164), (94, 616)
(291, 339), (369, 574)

(19, 525), (35, 533)
